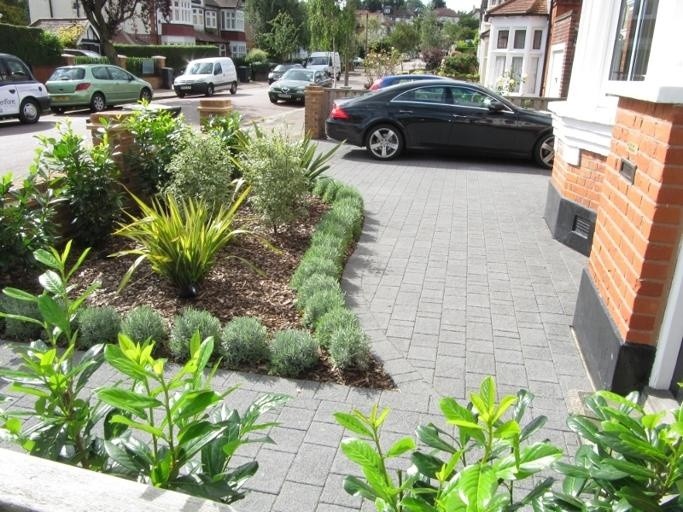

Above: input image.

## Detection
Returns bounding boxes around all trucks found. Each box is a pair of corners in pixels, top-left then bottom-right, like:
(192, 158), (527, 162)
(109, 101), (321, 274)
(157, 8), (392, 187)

(305, 51), (342, 80)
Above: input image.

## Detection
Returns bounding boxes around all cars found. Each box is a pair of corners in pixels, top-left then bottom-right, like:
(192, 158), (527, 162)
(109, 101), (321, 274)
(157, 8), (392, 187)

(268, 63), (333, 105)
(0, 52), (50, 124)
(325, 74), (554, 170)
(46, 65), (154, 111)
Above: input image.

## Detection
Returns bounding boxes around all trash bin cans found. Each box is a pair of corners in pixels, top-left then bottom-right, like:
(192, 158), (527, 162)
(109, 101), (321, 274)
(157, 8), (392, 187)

(161, 67), (173, 89)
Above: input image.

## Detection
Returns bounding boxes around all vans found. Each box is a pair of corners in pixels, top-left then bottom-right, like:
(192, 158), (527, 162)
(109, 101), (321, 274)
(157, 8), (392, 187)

(173, 57), (238, 96)
